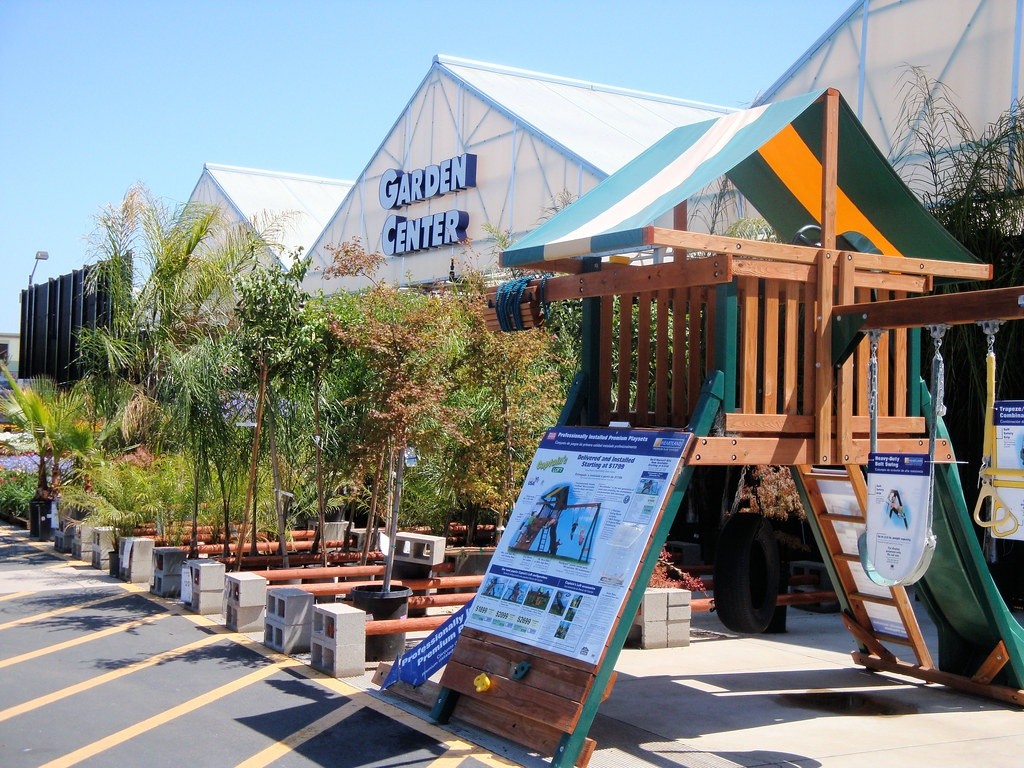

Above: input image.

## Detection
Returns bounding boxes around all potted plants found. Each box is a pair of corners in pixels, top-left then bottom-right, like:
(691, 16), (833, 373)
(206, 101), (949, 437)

(151, 236), (575, 661)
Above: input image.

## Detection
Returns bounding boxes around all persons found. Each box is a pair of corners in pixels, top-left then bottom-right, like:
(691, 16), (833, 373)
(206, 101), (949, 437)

(570, 519), (578, 540)
(578, 530), (584, 545)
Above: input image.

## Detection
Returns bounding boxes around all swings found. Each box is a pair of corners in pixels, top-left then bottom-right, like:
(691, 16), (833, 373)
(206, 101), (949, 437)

(857, 338), (946, 587)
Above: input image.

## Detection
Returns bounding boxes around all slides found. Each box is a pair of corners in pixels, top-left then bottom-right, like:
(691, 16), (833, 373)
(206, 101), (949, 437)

(860, 374), (1024, 689)
